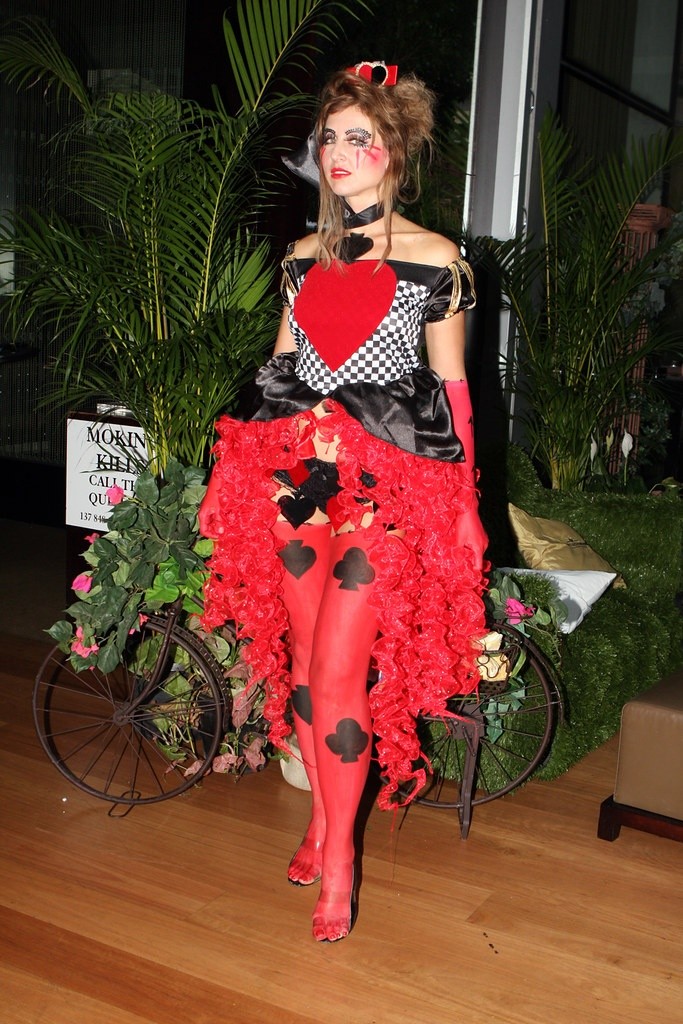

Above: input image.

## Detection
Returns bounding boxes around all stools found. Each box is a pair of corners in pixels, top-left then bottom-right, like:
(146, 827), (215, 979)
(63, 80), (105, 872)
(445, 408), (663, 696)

(598, 668), (682, 842)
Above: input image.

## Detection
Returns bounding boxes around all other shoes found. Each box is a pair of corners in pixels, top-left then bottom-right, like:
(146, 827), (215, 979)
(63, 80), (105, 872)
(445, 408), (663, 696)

(313, 862), (357, 942)
(288, 835), (324, 887)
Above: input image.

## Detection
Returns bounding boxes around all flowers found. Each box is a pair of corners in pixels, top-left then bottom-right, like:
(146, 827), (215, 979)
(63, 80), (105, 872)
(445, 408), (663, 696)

(41, 462), (247, 759)
(490, 584), (564, 661)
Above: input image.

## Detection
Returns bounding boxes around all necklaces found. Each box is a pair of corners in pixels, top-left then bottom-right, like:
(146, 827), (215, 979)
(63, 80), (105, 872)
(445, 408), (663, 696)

(342, 201), (384, 228)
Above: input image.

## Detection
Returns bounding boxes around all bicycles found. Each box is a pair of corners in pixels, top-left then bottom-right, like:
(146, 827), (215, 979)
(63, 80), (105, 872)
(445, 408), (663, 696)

(34, 527), (570, 839)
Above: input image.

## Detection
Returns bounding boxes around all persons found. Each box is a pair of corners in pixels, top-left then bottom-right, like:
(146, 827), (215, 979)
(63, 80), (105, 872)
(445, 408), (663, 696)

(199, 59), (493, 942)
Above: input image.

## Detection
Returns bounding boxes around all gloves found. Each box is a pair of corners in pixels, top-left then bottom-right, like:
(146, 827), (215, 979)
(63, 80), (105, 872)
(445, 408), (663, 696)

(439, 379), (489, 570)
(198, 459), (224, 539)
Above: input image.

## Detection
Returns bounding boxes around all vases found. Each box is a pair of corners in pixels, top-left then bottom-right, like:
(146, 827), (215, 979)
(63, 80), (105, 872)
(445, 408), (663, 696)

(194, 691), (313, 791)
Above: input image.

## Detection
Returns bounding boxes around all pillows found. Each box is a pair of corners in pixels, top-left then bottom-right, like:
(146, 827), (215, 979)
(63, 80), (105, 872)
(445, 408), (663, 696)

(494, 504), (626, 634)
